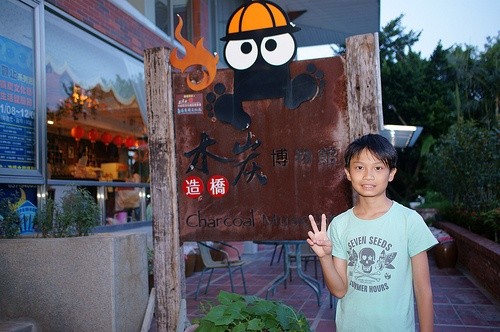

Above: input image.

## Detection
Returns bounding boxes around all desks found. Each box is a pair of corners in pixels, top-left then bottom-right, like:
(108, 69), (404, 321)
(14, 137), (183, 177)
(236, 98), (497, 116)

(253, 240), (322, 308)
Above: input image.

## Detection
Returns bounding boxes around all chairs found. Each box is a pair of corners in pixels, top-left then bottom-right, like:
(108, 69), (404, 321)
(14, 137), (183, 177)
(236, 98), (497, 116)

(270, 244), (321, 282)
(196, 241), (248, 299)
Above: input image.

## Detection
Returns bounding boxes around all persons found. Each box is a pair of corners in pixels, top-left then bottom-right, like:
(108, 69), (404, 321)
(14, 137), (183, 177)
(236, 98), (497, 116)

(306, 133), (440, 332)
(127, 136), (151, 222)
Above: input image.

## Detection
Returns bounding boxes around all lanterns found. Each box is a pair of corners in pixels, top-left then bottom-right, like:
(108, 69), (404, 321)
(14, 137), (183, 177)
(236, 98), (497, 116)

(71, 125), (135, 149)
